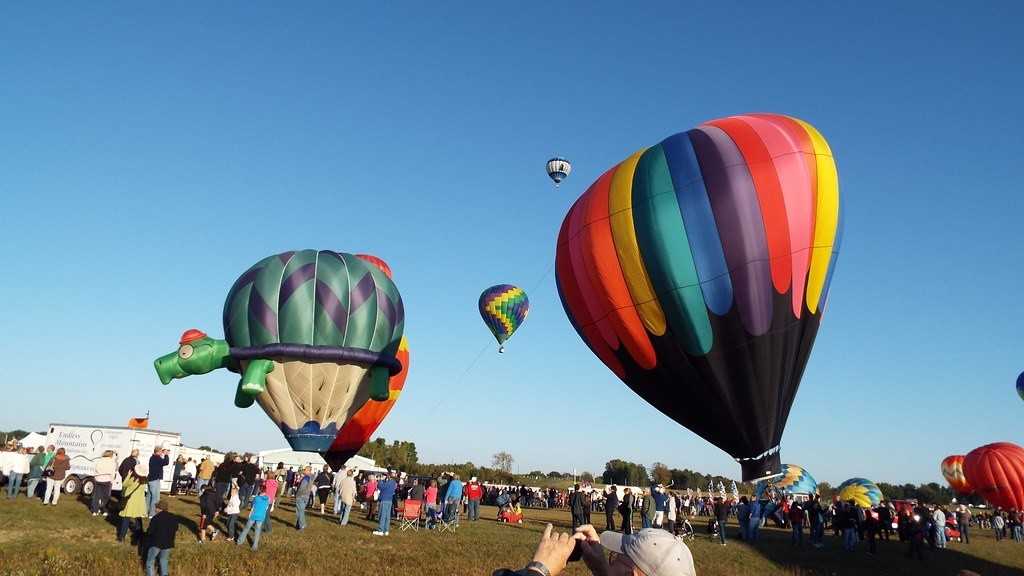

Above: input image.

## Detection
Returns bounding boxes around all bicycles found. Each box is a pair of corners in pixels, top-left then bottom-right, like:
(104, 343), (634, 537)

(672, 519), (695, 542)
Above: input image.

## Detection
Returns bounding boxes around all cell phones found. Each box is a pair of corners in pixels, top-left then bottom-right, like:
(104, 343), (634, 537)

(567, 539), (584, 564)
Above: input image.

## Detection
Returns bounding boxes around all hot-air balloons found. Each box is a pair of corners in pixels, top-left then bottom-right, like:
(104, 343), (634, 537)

(940, 455), (977, 505)
(836, 478), (885, 510)
(554, 115), (847, 521)
(478, 284), (530, 353)
(1015, 371), (1024, 401)
(963, 442), (1024, 519)
(756, 464), (823, 523)
(547, 158), (571, 188)
(153, 249), (411, 498)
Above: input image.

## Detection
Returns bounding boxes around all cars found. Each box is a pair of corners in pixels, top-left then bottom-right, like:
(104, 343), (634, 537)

(500, 511), (523, 524)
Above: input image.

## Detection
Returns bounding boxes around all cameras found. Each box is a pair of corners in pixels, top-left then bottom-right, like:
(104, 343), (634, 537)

(162, 449), (170, 453)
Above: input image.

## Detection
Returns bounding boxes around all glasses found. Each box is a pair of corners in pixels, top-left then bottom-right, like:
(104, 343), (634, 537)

(609, 551), (641, 576)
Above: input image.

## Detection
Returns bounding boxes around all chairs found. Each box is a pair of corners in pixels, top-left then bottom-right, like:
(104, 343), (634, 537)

(396, 500), (421, 532)
(432, 502), (458, 534)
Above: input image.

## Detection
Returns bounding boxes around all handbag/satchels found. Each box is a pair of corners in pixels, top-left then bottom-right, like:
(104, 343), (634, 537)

(275, 469), (285, 485)
(116, 496), (130, 510)
(374, 490), (379, 502)
(42, 457), (56, 476)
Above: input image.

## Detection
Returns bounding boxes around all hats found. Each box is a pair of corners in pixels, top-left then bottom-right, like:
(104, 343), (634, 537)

(134, 463), (150, 476)
(599, 527), (697, 576)
(155, 500), (170, 512)
(471, 477), (477, 482)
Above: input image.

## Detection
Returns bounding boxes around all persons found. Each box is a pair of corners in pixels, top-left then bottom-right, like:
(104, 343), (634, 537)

(197, 484), (220, 544)
(526, 561), (551, 576)
(463, 476), (483, 523)
(496, 493), (516, 521)
(444, 473), (462, 527)
(372, 472), (397, 536)
(566, 483), (589, 534)
(223, 488), (241, 541)
(118, 449), (140, 510)
(236, 485), (271, 552)
(313, 464), (334, 516)
(0, 438), (1024, 551)
(602, 486), (618, 531)
(90, 450), (117, 516)
(424, 479), (438, 529)
(492, 522), (698, 576)
(339, 470), (357, 527)
(142, 500), (179, 576)
(713, 496), (730, 547)
(292, 466), (313, 532)
(41, 447), (72, 506)
(144, 445), (169, 519)
(261, 472), (278, 532)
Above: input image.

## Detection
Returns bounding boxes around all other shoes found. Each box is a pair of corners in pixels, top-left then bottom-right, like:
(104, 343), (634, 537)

(225, 536), (234, 542)
(93, 509), (108, 517)
(372, 530), (389, 537)
(936, 544), (946, 548)
(814, 543), (824, 548)
(720, 543), (726, 547)
(6, 496), (18, 499)
(199, 529), (220, 544)
(1001, 539), (1004, 541)
